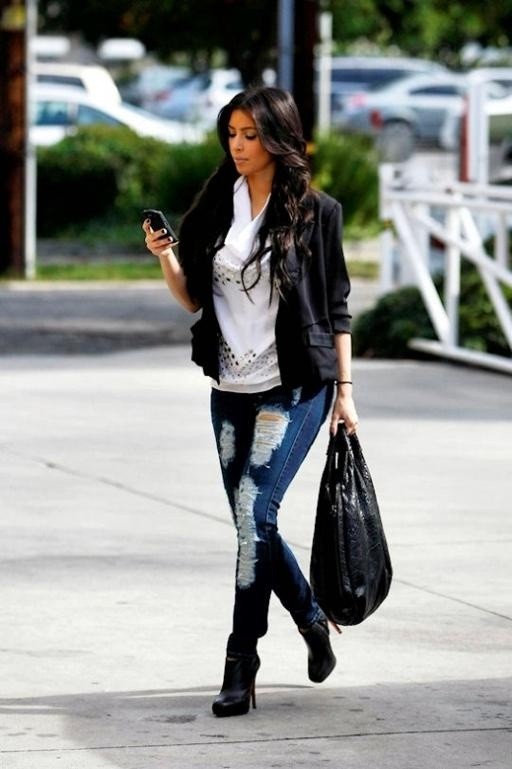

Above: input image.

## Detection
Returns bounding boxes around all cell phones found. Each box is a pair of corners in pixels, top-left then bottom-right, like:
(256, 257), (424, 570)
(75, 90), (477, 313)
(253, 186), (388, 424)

(143, 209), (179, 247)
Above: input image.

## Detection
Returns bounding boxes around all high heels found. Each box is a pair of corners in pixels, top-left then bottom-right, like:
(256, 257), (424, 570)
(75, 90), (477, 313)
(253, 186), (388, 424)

(212, 654), (260, 716)
(298, 613), (342, 683)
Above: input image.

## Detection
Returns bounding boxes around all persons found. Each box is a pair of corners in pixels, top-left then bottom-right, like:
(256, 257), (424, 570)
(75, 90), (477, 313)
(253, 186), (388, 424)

(140, 85), (361, 717)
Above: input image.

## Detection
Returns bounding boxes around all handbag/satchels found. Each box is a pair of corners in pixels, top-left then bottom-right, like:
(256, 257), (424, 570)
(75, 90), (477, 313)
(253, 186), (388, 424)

(311, 450), (392, 626)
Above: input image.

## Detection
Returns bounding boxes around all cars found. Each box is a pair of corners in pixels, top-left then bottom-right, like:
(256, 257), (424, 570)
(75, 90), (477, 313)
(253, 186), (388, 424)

(33, 33), (512, 165)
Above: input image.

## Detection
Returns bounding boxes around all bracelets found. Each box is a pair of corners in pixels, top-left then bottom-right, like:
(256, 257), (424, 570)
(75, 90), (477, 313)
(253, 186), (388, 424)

(337, 380), (352, 385)
